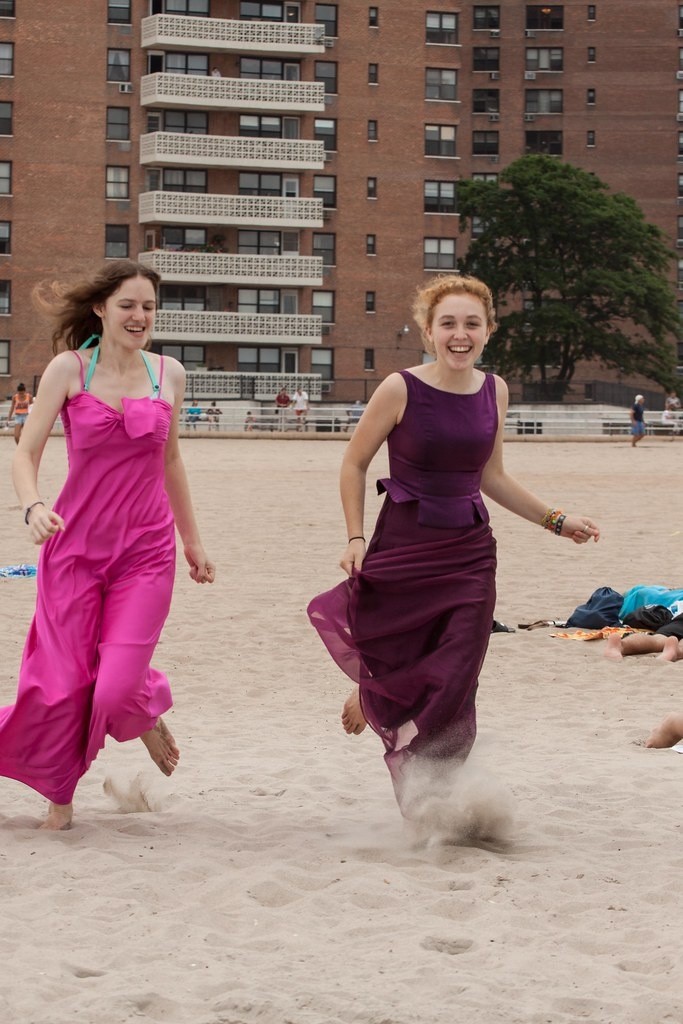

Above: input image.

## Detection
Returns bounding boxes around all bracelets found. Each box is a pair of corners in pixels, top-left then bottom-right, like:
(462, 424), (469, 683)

(348, 536), (365, 544)
(25, 501), (44, 525)
(541, 507), (566, 536)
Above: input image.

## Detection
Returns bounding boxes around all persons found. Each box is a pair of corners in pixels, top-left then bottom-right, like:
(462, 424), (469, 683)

(0, 260), (215, 829)
(6, 383), (34, 446)
(306, 274), (601, 825)
(662, 390), (682, 434)
(342, 400), (366, 432)
(243, 411), (256, 431)
(275, 388), (308, 432)
(205, 402), (223, 431)
(603, 613), (683, 664)
(645, 711), (683, 748)
(628, 394), (646, 447)
(187, 400), (202, 431)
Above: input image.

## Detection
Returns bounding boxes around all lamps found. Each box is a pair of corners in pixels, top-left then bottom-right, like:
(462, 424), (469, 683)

(397, 325), (410, 337)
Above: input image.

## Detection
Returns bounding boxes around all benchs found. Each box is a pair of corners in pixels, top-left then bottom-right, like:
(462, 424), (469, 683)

(337, 418), (367, 433)
(179, 409), (220, 430)
(602, 421), (675, 436)
(253, 416), (301, 431)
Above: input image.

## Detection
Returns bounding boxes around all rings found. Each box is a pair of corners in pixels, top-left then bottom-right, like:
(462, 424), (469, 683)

(582, 525), (589, 533)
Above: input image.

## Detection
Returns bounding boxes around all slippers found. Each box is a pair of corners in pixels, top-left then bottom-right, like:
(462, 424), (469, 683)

(490, 620), (515, 634)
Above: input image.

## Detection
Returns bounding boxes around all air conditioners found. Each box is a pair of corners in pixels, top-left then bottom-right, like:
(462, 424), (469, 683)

(676, 239), (683, 248)
(525, 31), (537, 38)
(677, 29), (683, 37)
(525, 72), (536, 79)
(489, 113), (499, 121)
(676, 113), (683, 121)
(676, 71), (683, 79)
(524, 114), (534, 121)
(119, 84), (135, 94)
(489, 30), (500, 37)
(326, 38), (334, 48)
(490, 73), (499, 80)
(324, 153), (333, 161)
(322, 384), (332, 393)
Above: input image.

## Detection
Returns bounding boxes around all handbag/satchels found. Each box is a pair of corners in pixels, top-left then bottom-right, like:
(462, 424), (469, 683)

(623, 604), (673, 631)
(565, 587), (625, 629)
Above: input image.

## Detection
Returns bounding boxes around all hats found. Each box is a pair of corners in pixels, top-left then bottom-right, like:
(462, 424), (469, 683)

(635, 395), (643, 404)
